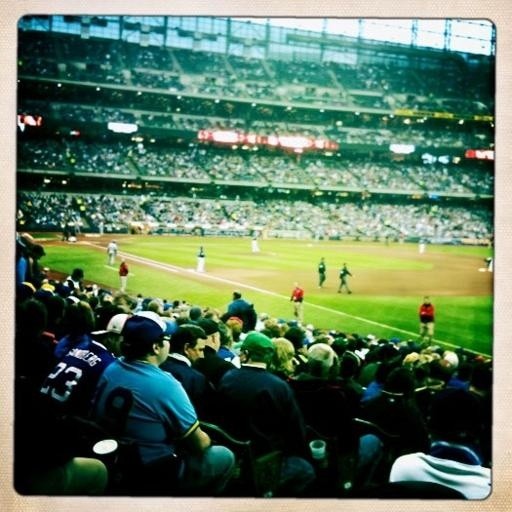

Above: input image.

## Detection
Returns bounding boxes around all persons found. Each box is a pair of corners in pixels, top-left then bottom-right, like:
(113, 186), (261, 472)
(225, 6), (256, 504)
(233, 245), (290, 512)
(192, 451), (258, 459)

(18, 231), (220, 495)
(252, 230), (261, 254)
(220, 288), (493, 501)
(16, 28), (493, 245)
(289, 282), (304, 321)
(337, 260), (353, 294)
(417, 236), (425, 255)
(483, 256), (493, 273)
(317, 256), (326, 290)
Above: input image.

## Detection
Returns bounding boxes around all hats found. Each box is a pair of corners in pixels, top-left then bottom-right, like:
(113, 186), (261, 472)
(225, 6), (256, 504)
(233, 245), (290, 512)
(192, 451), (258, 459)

(226, 317), (243, 335)
(121, 311), (177, 341)
(307, 343), (339, 366)
(233, 334), (275, 360)
(91, 314), (133, 337)
(196, 319), (226, 335)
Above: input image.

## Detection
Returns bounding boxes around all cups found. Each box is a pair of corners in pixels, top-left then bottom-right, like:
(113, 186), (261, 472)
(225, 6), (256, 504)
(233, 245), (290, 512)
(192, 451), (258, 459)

(309, 439), (327, 460)
